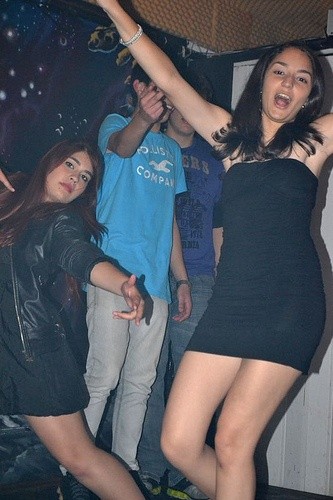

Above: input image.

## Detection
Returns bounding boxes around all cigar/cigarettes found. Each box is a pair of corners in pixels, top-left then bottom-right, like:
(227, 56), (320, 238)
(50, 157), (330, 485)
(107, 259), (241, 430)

(165, 104), (175, 112)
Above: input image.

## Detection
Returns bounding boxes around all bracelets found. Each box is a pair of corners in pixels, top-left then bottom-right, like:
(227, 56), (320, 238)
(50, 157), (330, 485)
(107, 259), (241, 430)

(119, 24), (143, 46)
(176, 280), (191, 289)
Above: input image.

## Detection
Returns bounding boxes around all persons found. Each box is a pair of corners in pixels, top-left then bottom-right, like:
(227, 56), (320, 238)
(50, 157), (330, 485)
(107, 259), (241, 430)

(90, 0), (333, 500)
(57, 64), (191, 500)
(136, 71), (223, 500)
(0, 138), (146, 500)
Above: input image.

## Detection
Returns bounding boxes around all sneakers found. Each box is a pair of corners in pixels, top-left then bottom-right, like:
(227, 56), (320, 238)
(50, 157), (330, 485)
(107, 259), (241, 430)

(143, 478), (162, 495)
(166, 477), (212, 500)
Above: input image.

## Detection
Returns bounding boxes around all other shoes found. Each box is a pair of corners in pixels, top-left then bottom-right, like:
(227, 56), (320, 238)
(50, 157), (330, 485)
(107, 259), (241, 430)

(59, 471), (93, 500)
